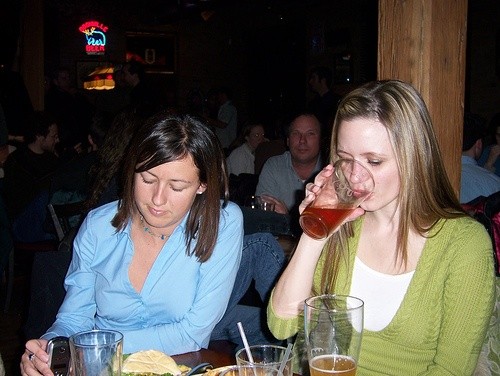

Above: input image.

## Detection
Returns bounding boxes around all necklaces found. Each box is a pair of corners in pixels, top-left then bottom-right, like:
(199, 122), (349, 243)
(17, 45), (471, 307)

(138, 215), (172, 241)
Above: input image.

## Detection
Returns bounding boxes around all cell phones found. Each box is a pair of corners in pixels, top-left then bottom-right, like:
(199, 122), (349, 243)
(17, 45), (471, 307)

(46, 336), (72, 376)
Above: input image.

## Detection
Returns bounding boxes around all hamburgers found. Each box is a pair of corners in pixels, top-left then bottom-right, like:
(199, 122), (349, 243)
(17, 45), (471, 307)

(121, 349), (181, 376)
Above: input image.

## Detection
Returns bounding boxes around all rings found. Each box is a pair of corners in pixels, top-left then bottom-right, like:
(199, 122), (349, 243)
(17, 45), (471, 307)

(28, 353), (35, 361)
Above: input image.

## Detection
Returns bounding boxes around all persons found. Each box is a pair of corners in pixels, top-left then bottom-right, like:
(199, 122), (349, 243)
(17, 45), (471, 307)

(19, 106), (245, 376)
(0, 44), (500, 358)
(266, 79), (497, 376)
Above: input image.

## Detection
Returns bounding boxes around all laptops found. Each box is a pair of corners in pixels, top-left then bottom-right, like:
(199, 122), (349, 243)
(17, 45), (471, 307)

(48, 200), (86, 241)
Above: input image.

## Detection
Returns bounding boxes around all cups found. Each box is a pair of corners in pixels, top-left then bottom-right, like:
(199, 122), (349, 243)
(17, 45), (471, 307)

(236, 345), (295, 376)
(219, 366), (283, 376)
(69, 330), (123, 376)
(300, 159), (374, 240)
(304, 295), (364, 376)
